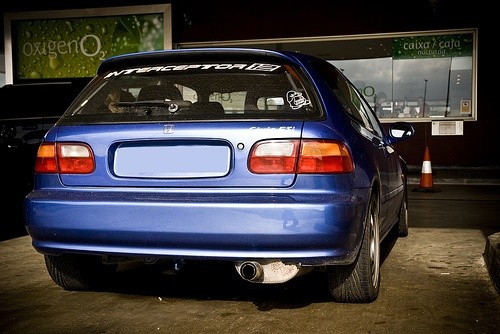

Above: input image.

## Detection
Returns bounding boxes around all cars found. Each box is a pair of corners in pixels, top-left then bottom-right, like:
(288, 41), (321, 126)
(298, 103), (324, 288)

(23, 42), (415, 306)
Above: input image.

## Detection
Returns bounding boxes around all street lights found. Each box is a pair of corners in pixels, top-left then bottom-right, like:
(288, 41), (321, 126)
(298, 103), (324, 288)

(423, 79), (428, 117)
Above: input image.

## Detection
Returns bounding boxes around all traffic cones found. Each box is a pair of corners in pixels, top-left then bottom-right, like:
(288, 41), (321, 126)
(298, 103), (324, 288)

(413, 144), (442, 192)
